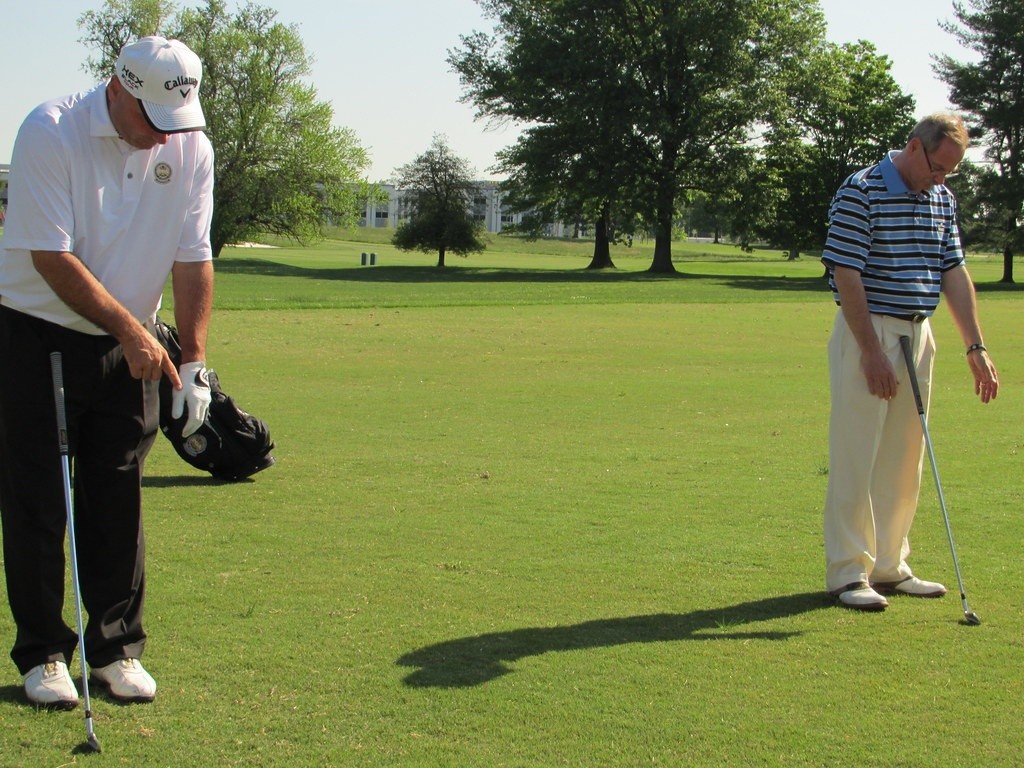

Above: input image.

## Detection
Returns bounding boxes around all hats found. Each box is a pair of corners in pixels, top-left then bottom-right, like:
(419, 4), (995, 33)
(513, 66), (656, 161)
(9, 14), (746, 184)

(114, 36), (208, 133)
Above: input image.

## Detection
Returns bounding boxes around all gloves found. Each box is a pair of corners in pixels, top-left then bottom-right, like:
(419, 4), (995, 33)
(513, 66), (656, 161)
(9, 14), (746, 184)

(171, 361), (212, 437)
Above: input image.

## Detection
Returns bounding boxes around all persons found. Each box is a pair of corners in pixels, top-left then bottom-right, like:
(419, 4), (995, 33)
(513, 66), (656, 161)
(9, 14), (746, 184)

(820, 113), (999, 608)
(0, 35), (214, 710)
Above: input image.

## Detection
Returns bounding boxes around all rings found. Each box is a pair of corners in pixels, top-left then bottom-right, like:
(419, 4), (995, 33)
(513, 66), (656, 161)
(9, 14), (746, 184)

(981, 384), (985, 388)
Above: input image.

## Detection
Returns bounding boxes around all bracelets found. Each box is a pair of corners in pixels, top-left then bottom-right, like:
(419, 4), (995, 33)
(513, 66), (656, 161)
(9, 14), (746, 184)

(967, 343), (987, 355)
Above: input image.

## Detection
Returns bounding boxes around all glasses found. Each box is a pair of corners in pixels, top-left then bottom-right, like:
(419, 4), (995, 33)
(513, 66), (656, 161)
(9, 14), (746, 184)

(917, 136), (960, 178)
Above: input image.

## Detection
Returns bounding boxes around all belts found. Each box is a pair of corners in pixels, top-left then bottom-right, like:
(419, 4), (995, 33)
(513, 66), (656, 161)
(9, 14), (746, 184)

(872, 310), (927, 326)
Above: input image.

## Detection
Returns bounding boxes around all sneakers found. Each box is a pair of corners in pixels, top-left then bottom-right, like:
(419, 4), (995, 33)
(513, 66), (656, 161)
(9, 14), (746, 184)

(24, 657), (80, 710)
(870, 576), (946, 597)
(831, 582), (889, 609)
(89, 658), (157, 701)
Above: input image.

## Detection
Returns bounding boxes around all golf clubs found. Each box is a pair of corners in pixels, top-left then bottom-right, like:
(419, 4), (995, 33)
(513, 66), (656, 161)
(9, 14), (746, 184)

(901, 335), (980, 626)
(49, 350), (103, 753)
